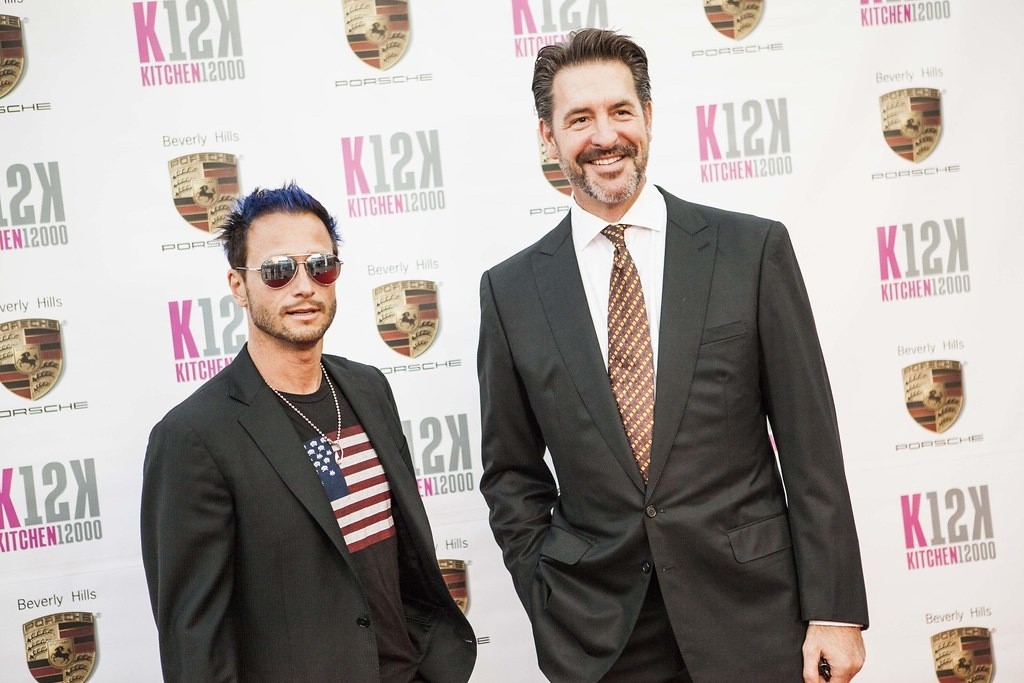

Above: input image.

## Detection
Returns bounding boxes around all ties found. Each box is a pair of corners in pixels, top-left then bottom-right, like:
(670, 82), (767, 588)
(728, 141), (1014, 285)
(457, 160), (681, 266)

(602, 222), (656, 479)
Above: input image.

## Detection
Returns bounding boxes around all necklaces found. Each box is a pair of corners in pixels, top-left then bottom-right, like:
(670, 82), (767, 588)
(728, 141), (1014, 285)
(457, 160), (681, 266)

(254, 363), (342, 456)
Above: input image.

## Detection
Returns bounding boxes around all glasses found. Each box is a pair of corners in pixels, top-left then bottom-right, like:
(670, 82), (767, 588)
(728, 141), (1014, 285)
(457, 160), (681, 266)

(235, 252), (345, 290)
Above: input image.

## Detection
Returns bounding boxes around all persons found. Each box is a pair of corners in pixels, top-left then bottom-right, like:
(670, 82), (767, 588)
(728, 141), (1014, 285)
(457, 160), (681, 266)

(476, 25), (868, 683)
(140, 178), (477, 683)
